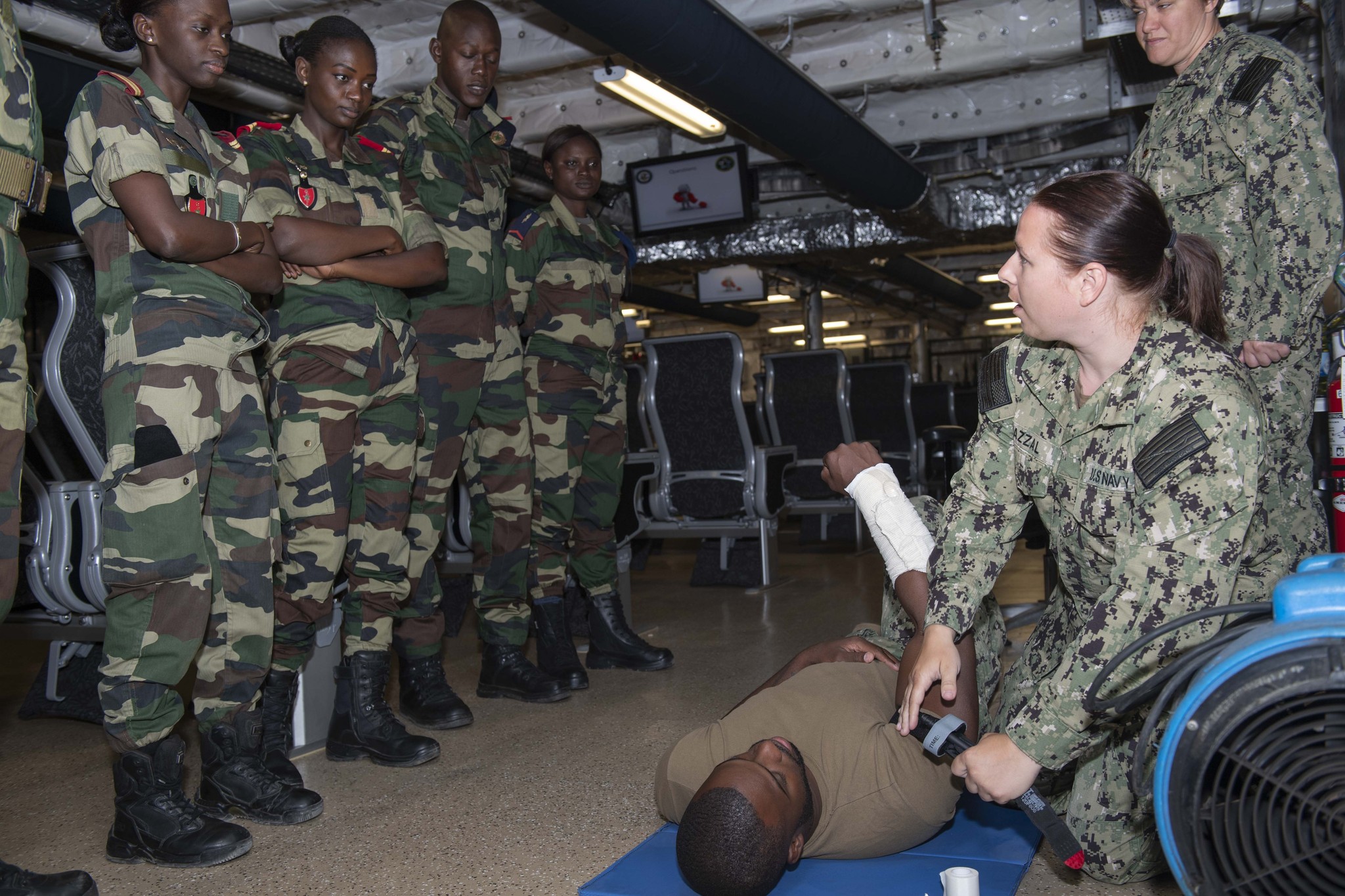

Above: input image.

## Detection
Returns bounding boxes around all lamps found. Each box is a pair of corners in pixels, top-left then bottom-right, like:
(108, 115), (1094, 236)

(592, 50), (727, 142)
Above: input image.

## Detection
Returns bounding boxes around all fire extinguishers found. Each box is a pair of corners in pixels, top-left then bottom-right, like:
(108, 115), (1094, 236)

(1312, 380), (1345, 553)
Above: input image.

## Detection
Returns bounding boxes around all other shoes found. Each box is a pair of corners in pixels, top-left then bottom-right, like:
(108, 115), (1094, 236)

(0, 858), (98, 896)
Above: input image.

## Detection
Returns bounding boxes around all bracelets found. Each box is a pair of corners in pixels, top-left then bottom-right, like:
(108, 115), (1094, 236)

(224, 220), (241, 255)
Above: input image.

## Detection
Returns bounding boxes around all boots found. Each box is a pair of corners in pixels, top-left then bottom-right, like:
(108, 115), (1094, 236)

(105, 734), (253, 869)
(476, 638), (572, 704)
(256, 668), (305, 789)
(397, 654), (476, 730)
(326, 650), (441, 768)
(194, 707), (324, 826)
(533, 595), (590, 690)
(585, 589), (675, 671)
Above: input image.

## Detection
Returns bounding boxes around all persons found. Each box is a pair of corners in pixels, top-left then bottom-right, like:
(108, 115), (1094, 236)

(652, 439), (1012, 896)
(235, 14), (449, 789)
(501, 124), (674, 693)
(1110, 0), (1345, 559)
(354, 0), (571, 732)
(892, 174), (1332, 884)
(63, 0), (327, 866)
(0, 0), (98, 896)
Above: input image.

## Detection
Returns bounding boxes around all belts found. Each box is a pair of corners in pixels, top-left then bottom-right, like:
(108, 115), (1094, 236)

(0, 149), (53, 216)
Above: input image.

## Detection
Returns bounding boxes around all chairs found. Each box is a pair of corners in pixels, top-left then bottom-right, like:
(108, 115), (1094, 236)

(17, 235), (966, 700)
